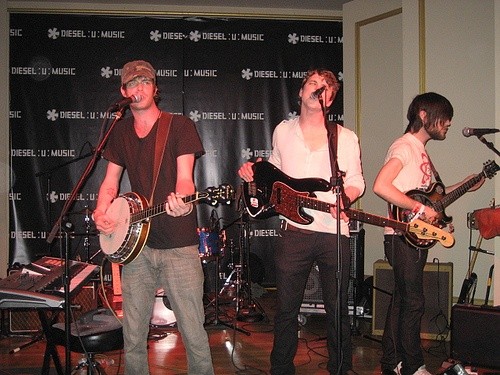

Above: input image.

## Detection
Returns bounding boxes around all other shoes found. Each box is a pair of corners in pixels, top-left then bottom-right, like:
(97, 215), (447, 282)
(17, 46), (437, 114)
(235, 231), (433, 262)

(330, 368), (359, 375)
(413, 364), (432, 375)
(394, 361), (401, 375)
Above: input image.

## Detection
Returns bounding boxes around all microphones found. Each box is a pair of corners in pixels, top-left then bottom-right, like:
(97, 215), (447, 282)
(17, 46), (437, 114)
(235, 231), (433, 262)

(310, 85), (326, 99)
(462, 128), (500, 137)
(110, 94), (142, 109)
(88, 141), (95, 155)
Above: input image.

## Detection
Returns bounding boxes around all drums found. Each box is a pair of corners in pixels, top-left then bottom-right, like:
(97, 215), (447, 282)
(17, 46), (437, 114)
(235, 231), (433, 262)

(197, 227), (225, 263)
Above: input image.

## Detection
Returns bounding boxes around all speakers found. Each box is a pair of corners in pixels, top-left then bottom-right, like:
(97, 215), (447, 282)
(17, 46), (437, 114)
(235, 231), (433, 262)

(298, 229), (366, 314)
(8, 281), (97, 334)
(450, 303), (500, 368)
(371, 259), (455, 341)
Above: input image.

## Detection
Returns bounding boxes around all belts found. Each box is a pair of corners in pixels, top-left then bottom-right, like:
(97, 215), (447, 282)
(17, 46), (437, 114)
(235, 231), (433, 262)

(282, 220), (315, 234)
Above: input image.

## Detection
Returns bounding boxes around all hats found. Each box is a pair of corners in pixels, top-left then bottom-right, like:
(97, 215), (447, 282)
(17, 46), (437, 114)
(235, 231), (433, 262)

(120, 60), (156, 87)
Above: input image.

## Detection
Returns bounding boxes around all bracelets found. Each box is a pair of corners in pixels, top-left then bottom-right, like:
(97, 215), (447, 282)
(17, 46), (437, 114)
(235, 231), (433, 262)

(181, 203), (193, 216)
(412, 203), (425, 221)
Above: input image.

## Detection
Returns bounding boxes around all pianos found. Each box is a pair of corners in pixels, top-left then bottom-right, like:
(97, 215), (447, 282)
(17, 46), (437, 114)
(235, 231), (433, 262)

(0, 256), (101, 375)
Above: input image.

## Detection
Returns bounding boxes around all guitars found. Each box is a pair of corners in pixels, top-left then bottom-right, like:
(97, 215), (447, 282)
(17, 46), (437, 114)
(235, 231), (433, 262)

(457, 198), (495, 304)
(393, 159), (500, 249)
(98, 183), (236, 264)
(242, 160), (455, 249)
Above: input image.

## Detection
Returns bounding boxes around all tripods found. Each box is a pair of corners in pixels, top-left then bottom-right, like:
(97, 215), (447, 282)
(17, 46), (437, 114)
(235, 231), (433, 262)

(202, 205), (263, 336)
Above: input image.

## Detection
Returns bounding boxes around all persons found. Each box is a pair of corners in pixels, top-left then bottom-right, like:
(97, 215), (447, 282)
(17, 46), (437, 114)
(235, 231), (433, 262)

(372, 92), (485, 375)
(238, 67), (365, 375)
(92, 61), (214, 375)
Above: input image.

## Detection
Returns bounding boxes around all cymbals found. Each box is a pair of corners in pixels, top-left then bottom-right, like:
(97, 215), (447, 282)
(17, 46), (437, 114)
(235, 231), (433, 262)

(67, 209), (93, 214)
